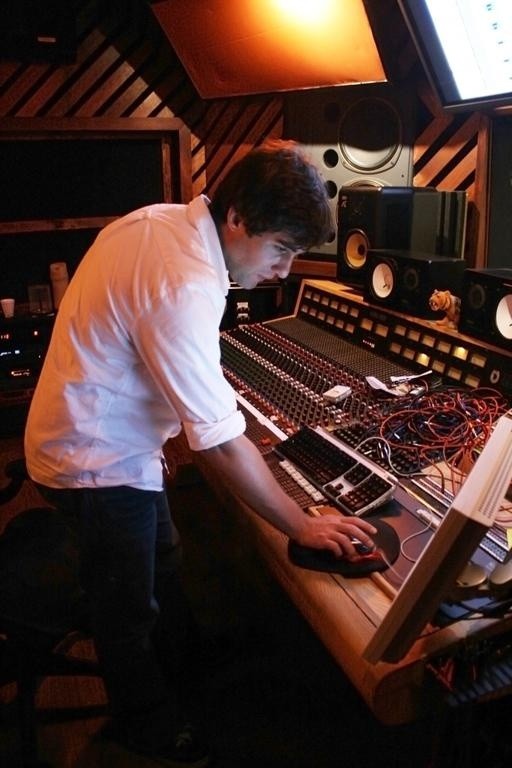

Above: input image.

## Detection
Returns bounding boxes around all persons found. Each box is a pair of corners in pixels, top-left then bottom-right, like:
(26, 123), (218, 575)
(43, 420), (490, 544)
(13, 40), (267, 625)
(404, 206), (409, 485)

(18, 146), (378, 761)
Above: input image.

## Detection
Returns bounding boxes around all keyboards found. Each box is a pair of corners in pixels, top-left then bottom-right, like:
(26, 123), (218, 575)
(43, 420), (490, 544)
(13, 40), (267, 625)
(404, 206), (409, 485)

(272, 425), (397, 517)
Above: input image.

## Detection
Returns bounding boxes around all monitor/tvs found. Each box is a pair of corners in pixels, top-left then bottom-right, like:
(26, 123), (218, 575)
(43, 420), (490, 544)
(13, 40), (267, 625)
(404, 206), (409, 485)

(140, 1), (399, 103)
(361, 414), (512, 666)
(398, 0), (512, 116)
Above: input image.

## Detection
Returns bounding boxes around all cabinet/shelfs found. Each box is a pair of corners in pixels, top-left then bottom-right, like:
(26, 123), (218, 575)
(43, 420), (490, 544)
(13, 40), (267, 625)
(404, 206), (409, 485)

(1, 116), (192, 324)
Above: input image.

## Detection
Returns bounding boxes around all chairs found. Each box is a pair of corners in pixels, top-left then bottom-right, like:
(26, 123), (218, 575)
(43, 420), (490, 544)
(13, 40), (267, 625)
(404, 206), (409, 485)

(1, 457), (110, 723)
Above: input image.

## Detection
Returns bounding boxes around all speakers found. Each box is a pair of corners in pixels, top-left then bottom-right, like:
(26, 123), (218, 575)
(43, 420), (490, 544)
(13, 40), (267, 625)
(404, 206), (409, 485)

(283, 72), (415, 260)
(457, 267), (512, 352)
(336, 186), (439, 287)
(0, 0), (77, 65)
(363, 249), (466, 321)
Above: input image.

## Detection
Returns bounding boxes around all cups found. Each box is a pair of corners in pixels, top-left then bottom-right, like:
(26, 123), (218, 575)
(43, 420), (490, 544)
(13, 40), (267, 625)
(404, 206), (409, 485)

(50, 262), (68, 309)
(1, 298), (16, 318)
(28, 284), (53, 314)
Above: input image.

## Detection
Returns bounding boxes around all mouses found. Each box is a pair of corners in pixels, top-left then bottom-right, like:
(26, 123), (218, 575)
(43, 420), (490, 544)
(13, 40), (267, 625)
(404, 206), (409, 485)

(348, 535), (375, 554)
